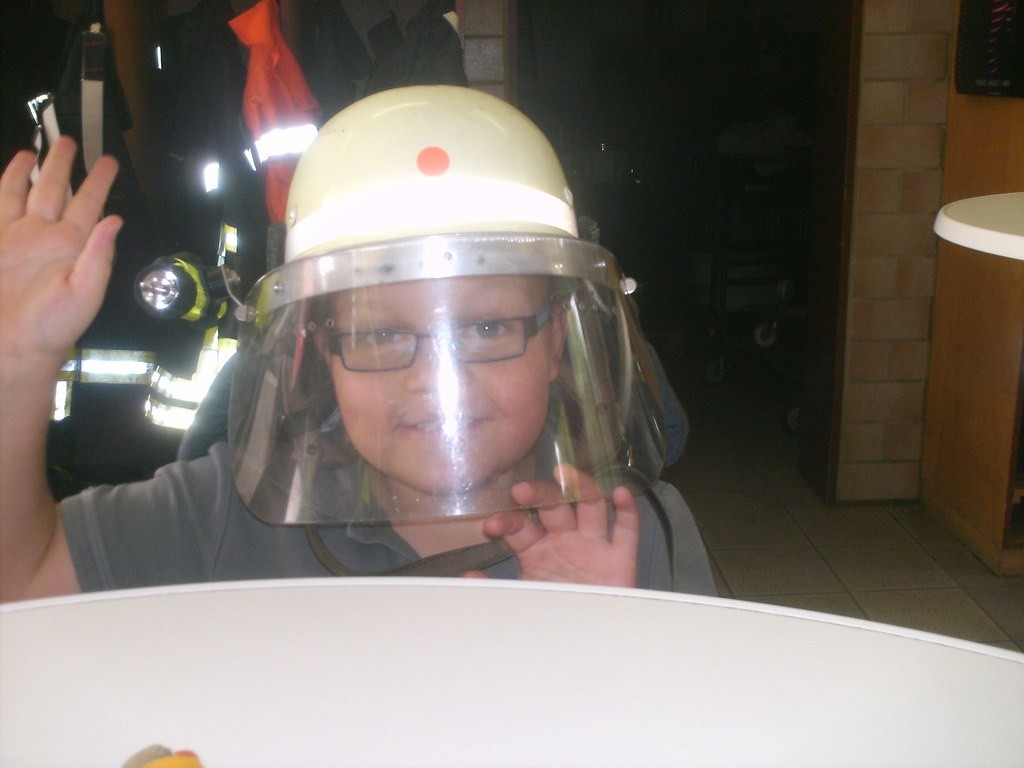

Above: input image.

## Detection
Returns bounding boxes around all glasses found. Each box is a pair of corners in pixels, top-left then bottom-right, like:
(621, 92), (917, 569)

(318, 301), (555, 373)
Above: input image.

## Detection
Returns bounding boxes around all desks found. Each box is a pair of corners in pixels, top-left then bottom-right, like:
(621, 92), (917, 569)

(934, 191), (1024, 261)
(0, 575), (1024, 768)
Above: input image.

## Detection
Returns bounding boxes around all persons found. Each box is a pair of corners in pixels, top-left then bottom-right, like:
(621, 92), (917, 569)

(0, 0), (468, 511)
(0, 83), (719, 602)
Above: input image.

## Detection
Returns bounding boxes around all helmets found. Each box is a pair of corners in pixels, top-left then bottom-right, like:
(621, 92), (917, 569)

(250, 84), (623, 327)
